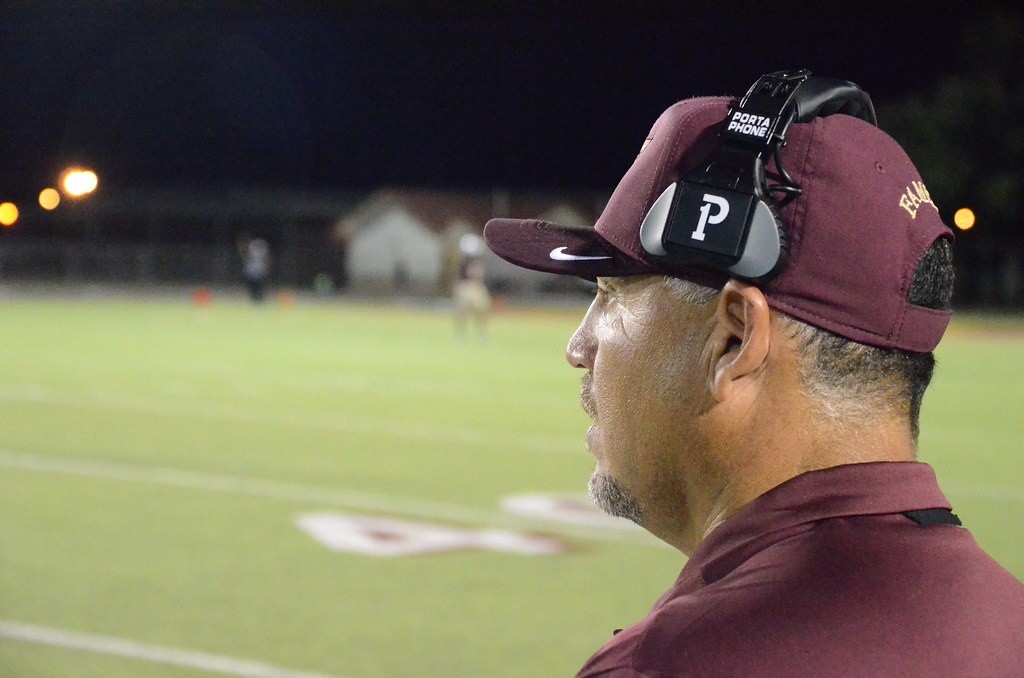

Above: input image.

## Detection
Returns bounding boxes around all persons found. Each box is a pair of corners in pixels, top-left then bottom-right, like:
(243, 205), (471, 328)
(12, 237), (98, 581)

(483, 96), (1024, 678)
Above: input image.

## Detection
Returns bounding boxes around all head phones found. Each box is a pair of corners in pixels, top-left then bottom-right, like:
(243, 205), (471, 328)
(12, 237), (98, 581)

(660, 68), (877, 263)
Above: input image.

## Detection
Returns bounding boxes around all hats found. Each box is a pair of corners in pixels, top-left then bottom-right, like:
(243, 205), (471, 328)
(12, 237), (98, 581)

(485, 98), (954, 351)
